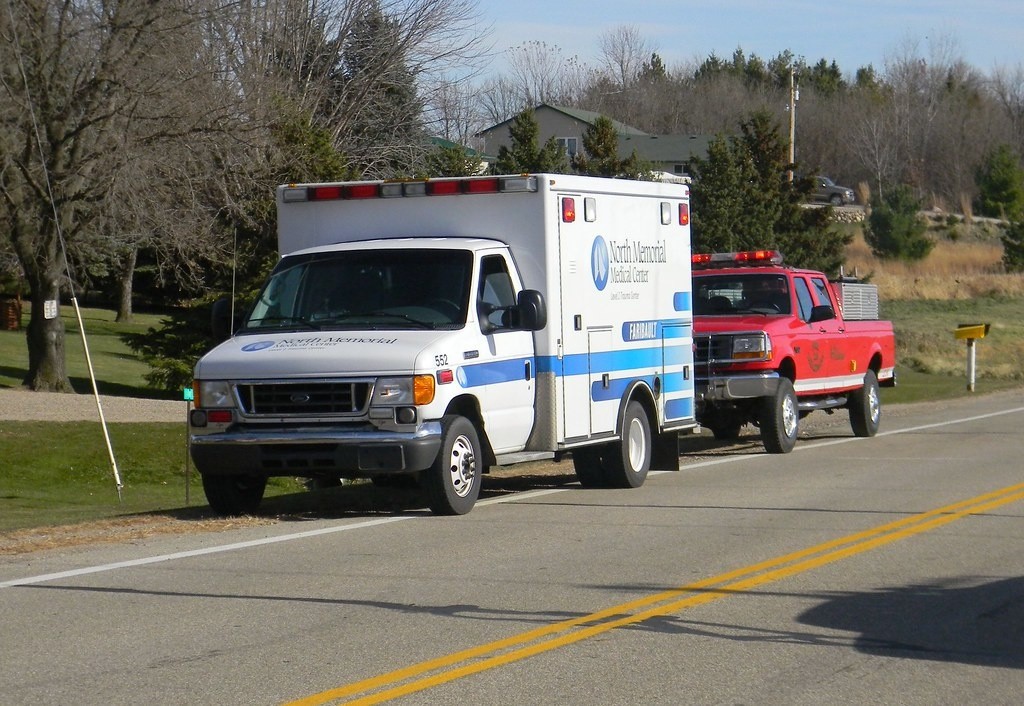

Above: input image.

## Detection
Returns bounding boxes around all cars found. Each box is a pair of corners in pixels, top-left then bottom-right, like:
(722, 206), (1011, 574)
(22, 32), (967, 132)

(810, 176), (854, 207)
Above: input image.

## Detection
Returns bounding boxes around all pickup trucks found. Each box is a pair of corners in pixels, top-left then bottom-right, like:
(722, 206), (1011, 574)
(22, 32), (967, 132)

(692, 252), (897, 455)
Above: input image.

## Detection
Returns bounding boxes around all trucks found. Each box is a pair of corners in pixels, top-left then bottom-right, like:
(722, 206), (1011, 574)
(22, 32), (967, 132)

(187, 170), (698, 515)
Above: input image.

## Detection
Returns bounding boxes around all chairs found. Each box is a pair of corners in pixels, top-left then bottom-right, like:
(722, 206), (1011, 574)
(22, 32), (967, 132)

(707, 296), (732, 310)
